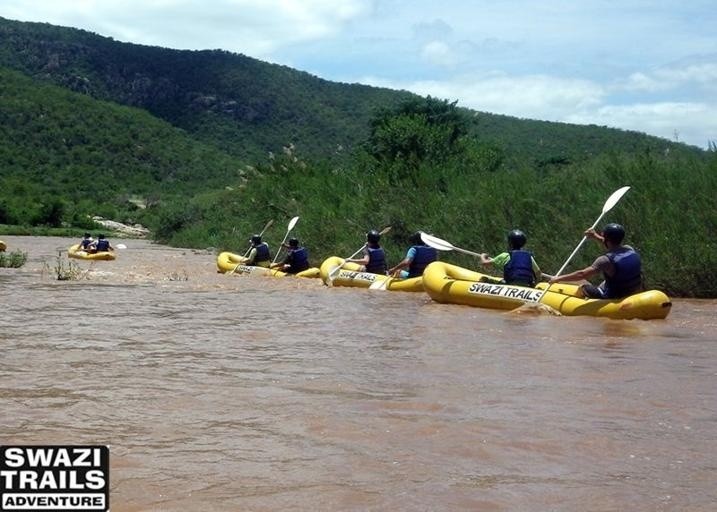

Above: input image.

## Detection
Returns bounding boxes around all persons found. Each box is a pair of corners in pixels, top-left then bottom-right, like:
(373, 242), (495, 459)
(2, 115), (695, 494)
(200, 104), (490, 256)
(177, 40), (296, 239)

(480, 228), (542, 288)
(270, 237), (310, 273)
(239, 234), (270, 266)
(74, 231), (97, 253)
(548, 222), (642, 299)
(345, 229), (388, 276)
(87, 233), (115, 254)
(388, 232), (438, 279)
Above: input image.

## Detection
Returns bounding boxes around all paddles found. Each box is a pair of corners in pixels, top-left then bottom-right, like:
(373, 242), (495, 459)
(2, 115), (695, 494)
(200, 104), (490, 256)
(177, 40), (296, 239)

(56, 246), (89, 252)
(89, 244), (129, 249)
(267, 216), (300, 272)
(327, 226), (390, 280)
(421, 233), (494, 261)
(369, 266), (394, 290)
(538, 186), (629, 303)
(230, 220), (273, 275)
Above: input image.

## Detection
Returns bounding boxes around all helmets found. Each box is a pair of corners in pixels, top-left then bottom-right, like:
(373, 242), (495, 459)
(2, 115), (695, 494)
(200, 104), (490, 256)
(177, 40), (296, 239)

(409, 232), (421, 240)
(85, 231), (105, 238)
(289, 238), (298, 245)
(366, 230), (380, 242)
(601, 223), (626, 241)
(251, 234), (261, 242)
(507, 229), (526, 246)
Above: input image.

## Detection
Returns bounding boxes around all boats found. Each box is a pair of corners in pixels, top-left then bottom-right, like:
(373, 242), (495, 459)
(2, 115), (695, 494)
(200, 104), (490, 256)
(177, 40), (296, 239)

(0, 239), (6, 252)
(215, 250), (320, 278)
(68, 243), (118, 261)
(321, 254), (427, 293)
(422, 261), (672, 319)
